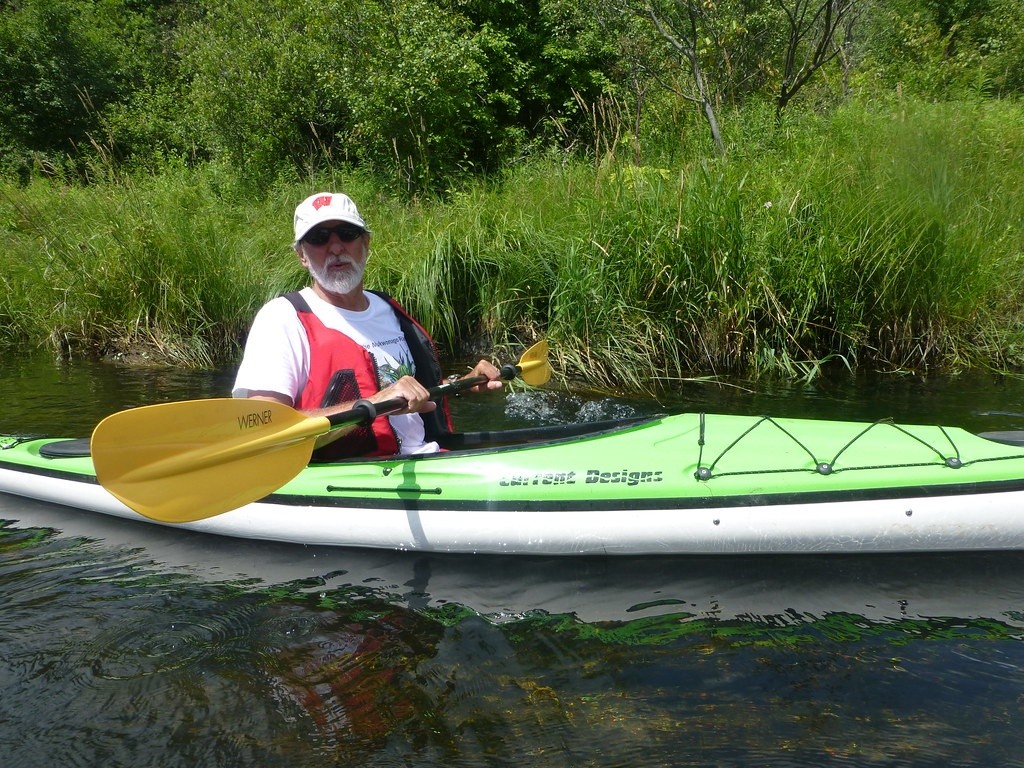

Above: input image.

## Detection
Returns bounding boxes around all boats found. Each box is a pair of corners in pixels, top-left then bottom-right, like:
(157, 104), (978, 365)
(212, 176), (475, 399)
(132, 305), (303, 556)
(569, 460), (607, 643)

(2, 409), (1023, 554)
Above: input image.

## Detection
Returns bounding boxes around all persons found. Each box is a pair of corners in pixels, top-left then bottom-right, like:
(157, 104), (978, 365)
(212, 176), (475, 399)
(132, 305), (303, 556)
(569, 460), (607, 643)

(230, 192), (503, 459)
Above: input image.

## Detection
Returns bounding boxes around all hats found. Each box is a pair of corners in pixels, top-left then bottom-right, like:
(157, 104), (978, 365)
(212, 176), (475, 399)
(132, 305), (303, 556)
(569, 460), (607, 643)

(293, 192), (366, 241)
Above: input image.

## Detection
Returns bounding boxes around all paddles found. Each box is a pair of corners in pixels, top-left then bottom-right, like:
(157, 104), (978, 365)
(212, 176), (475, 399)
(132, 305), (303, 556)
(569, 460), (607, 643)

(91, 340), (552, 524)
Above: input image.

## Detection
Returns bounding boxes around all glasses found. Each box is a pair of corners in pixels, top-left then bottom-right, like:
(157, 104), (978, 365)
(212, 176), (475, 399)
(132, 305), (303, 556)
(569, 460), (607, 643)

(302, 222), (363, 248)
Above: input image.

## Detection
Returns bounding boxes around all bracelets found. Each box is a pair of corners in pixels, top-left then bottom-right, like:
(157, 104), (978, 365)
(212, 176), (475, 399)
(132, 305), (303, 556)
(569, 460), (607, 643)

(447, 374), (462, 382)
